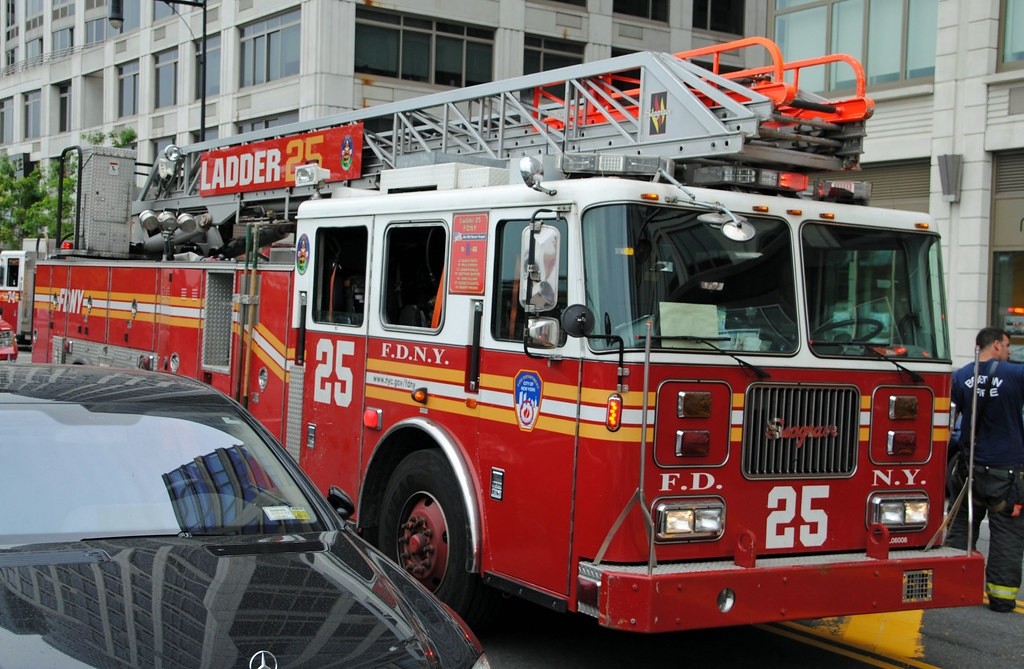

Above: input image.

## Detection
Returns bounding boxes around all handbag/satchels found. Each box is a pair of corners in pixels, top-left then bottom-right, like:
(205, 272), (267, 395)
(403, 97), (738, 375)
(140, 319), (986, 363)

(973, 464), (1015, 505)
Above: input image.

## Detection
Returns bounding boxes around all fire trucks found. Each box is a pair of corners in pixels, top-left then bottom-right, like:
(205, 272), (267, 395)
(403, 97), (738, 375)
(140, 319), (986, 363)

(0, 35), (987, 636)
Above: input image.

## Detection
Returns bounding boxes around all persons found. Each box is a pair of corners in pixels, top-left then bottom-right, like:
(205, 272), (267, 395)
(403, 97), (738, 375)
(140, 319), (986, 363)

(943, 326), (1024, 612)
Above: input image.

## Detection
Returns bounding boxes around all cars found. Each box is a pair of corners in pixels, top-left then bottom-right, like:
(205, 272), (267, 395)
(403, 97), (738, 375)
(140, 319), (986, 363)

(0, 364), (493, 669)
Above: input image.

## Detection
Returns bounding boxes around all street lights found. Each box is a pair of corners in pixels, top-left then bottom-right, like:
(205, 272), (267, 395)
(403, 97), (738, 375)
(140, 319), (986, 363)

(109, 0), (206, 142)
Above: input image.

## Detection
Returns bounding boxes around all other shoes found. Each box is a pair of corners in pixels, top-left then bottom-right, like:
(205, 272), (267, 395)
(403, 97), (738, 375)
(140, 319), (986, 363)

(990, 602), (1011, 611)
